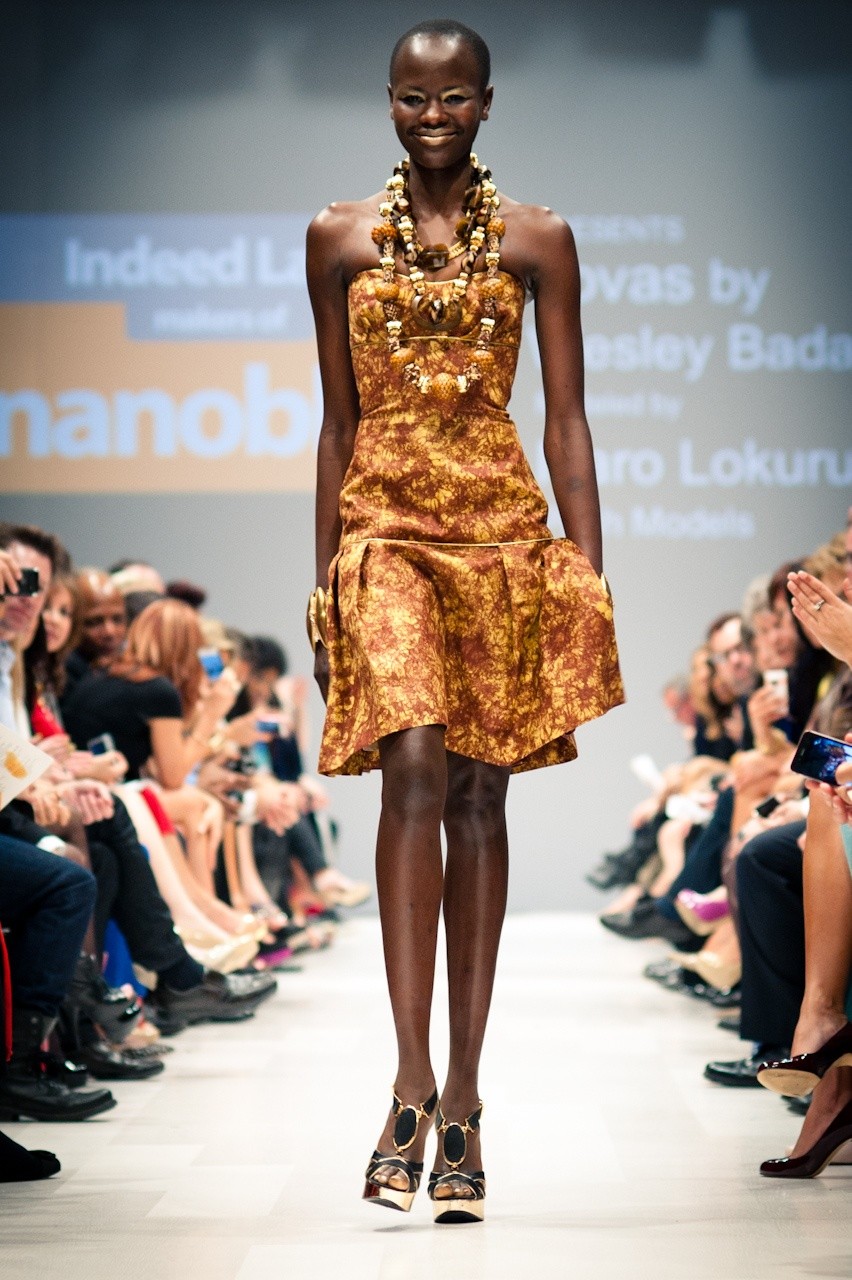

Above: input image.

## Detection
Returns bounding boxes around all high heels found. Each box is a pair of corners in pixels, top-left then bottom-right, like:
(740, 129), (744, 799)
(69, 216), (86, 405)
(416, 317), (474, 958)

(755, 1019), (851, 1099)
(361, 1084), (441, 1214)
(760, 1101), (851, 1179)
(427, 1097), (486, 1223)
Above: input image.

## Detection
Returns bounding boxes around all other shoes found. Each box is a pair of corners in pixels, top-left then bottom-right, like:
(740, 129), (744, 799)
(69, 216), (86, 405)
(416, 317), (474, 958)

(582, 814), (852, 1034)
(0, 1131), (62, 1184)
(1, 868), (374, 1057)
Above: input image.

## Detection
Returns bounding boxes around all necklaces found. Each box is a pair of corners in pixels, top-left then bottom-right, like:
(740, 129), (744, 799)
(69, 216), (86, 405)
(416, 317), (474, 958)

(369, 152), (506, 400)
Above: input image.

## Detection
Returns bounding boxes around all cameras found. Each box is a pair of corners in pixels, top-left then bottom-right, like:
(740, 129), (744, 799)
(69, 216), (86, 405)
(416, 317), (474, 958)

(87, 732), (115, 756)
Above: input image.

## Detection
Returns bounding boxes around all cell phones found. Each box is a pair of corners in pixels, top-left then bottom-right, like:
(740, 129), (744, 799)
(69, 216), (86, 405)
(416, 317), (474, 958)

(788, 729), (852, 787)
(4, 567), (40, 597)
(763, 668), (789, 718)
(196, 647), (224, 683)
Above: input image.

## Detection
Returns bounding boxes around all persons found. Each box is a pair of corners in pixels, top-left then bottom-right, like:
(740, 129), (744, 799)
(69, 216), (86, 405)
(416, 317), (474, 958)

(0, 524), (372, 1183)
(306, 18), (625, 1233)
(577, 507), (852, 1180)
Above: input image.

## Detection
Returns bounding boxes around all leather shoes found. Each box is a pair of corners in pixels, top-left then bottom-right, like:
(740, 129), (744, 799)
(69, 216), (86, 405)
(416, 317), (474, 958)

(704, 1038), (813, 1116)
(1, 1064), (117, 1123)
(65, 1040), (164, 1086)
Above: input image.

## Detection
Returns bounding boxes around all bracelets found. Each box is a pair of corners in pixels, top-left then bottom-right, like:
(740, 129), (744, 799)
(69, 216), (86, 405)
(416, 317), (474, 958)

(305, 585), (328, 653)
